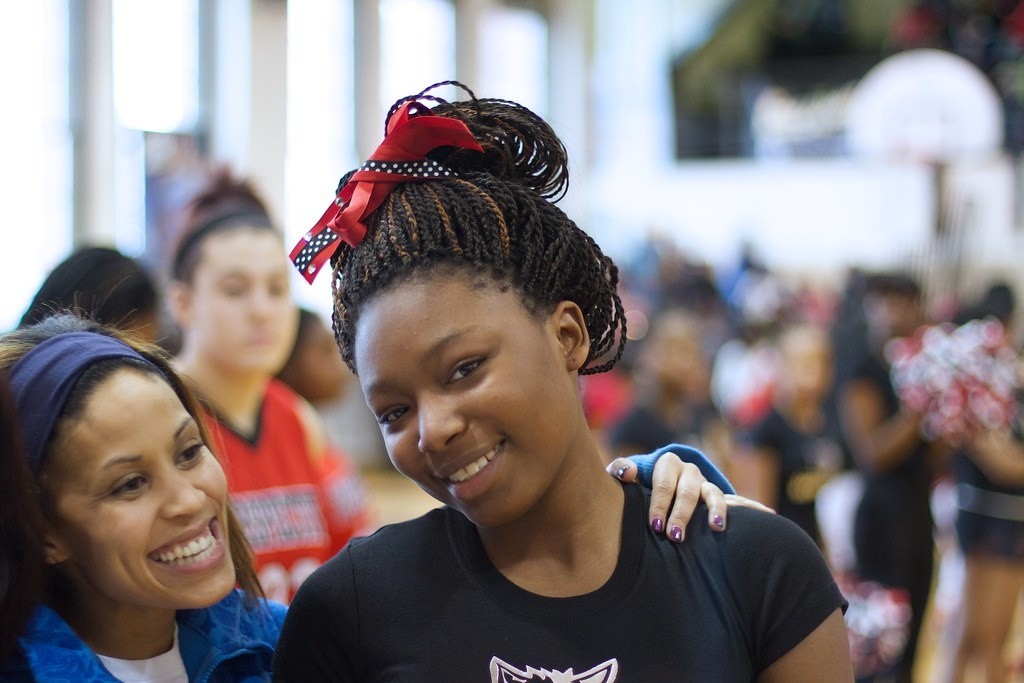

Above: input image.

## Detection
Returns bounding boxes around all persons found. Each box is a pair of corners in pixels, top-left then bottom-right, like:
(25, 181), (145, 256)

(267, 80), (856, 683)
(582, 235), (1024, 683)
(0, 172), (775, 683)
